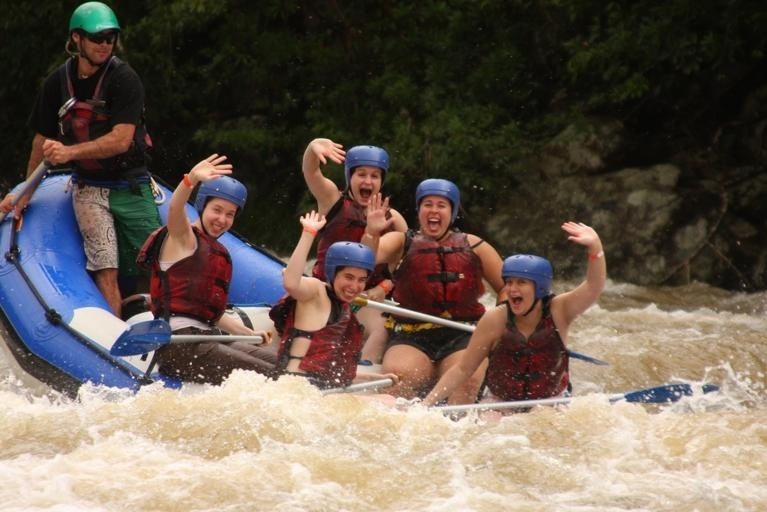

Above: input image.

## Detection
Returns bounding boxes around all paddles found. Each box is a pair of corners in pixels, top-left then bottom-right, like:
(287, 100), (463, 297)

(353, 294), (608, 365)
(426, 381), (719, 413)
(110, 318), (272, 357)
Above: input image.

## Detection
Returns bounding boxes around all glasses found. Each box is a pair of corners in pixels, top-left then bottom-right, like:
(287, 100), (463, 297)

(76, 29), (117, 46)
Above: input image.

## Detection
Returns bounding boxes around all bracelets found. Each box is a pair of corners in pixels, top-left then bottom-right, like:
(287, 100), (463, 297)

(302, 227), (317, 235)
(379, 282), (390, 295)
(586, 251), (604, 259)
(182, 174), (192, 189)
(364, 227), (380, 240)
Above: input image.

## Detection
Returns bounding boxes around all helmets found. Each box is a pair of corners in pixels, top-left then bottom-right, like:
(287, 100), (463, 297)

(501, 254), (553, 299)
(344, 145), (390, 192)
(193, 175), (247, 214)
(68, 0), (121, 35)
(415, 178), (461, 223)
(324, 240), (376, 283)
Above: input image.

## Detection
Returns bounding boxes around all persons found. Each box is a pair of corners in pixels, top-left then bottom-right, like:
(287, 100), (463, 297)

(0, 1), (162, 319)
(360, 177), (508, 407)
(270, 209), (375, 396)
(136, 153), (278, 387)
(301, 137), (408, 364)
(419, 221), (607, 421)
